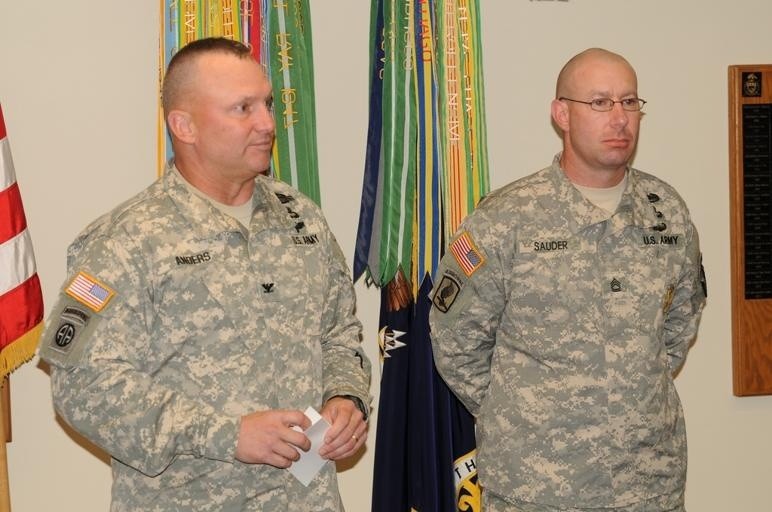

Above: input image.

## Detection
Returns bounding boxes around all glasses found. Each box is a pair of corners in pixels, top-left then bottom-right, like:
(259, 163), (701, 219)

(559, 96), (646, 112)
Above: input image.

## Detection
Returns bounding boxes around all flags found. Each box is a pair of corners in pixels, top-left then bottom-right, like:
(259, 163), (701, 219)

(1, 104), (44, 394)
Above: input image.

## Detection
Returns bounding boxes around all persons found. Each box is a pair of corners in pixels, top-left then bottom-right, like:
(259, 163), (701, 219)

(429, 49), (707, 511)
(40, 38), (374, 511)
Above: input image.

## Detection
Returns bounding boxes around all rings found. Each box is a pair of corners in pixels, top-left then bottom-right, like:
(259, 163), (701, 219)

(351, 433), (359, 441)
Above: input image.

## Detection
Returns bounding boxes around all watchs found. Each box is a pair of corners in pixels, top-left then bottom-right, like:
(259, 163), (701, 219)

(335, 392), (369, 422)
